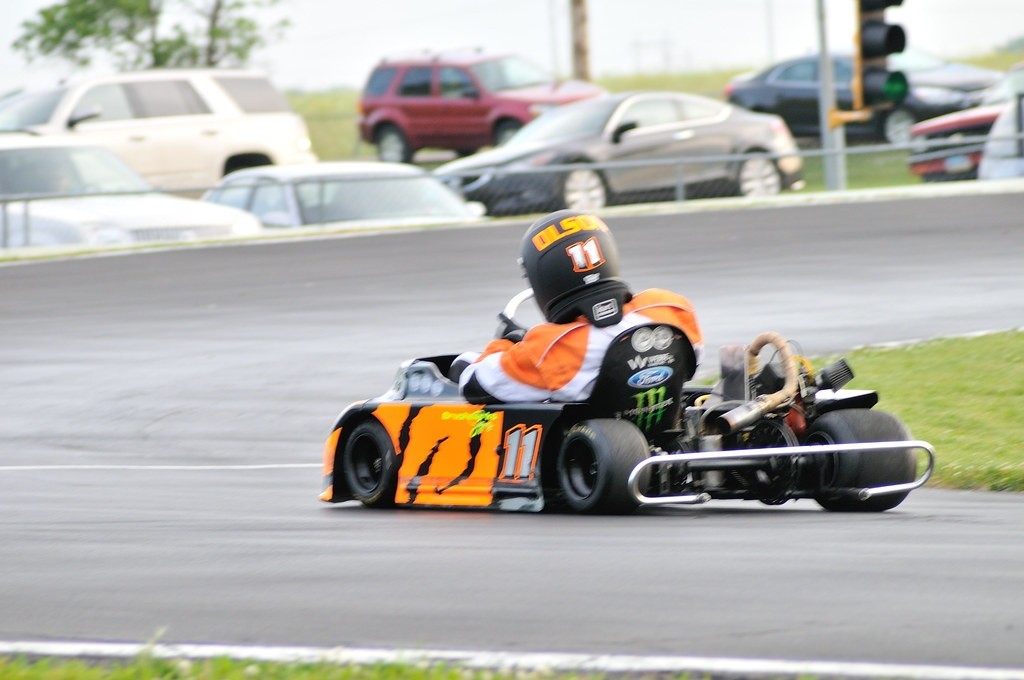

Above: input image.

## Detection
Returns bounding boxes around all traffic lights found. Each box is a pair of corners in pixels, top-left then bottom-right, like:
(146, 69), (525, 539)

(854, 2), (907, 107)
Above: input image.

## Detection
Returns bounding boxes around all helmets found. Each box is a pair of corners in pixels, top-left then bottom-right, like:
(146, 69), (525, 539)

(519, 211), (633, 324)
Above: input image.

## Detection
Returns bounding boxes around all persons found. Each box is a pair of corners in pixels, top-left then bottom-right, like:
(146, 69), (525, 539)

(459, 208), (704, 406)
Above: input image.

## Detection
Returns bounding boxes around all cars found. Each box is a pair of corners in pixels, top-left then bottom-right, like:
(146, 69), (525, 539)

(725, 52), (1024, 145)
(431, 90), (806, 219)
(0, 135), (263, 256)
(201, 161), (484, 231)
(907, 69), (1024, 184)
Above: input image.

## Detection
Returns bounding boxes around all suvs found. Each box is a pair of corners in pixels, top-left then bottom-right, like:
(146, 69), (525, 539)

(0, 67), (320, 193)
(356, 50), (607, 164)
